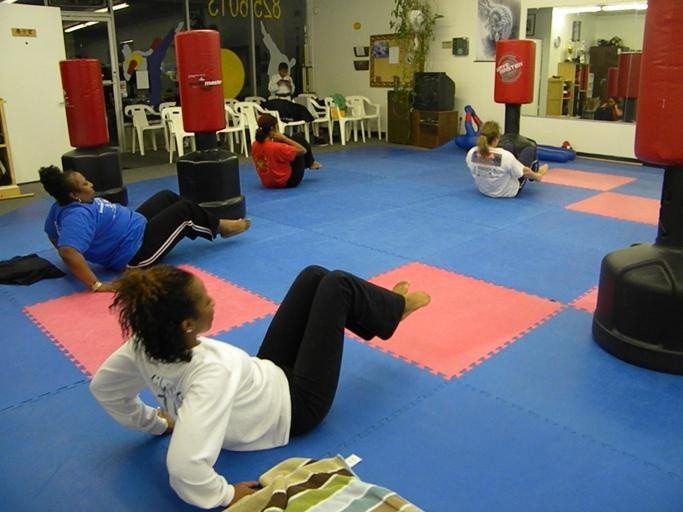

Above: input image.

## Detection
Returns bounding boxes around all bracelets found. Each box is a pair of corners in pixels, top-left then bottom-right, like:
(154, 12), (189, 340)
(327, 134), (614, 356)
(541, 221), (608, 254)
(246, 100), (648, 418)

(89, 280), (103, 292)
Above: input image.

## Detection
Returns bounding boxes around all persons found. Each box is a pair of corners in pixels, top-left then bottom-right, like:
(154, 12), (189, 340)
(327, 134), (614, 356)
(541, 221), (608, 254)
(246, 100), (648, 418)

(252, 113), (321, 189)
(465, 120), (549, 200)
(593, 96), (623, 121)
(85, 262), (431, 510)
(268, 62), (296, 103)
(39, 160), (252, 293)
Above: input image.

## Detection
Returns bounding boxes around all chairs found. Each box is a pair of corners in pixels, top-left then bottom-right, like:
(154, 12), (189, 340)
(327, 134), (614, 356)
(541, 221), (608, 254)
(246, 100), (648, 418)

(215, 104), (249, 158)
(347, 94), (381, 146)
(337, 97), (366, 145)
(294, 93), (334, 147)
(323, 96), (352, 141)
(124, 103), (163, 156)
(223, 97), (241, 144)
(233, 101), (281, 153)
(267, 95), (311, 144)
(162, 106), (197, 158)
(159, 100), (189, 152)
(246, 95), (267, 105)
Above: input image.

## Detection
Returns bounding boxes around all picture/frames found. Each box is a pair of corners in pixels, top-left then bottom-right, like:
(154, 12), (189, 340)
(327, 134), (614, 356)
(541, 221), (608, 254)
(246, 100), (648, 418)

(526, 13), (535, 36)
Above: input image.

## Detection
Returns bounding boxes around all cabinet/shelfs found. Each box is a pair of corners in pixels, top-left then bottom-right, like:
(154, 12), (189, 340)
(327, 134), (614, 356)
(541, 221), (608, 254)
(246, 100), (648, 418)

(547, 78), (572, 117)
(557, 60), (589, 118)
(590, 44), (630, 99)
(0, 98), (21, 200)
(411, 109), (458, 149)
